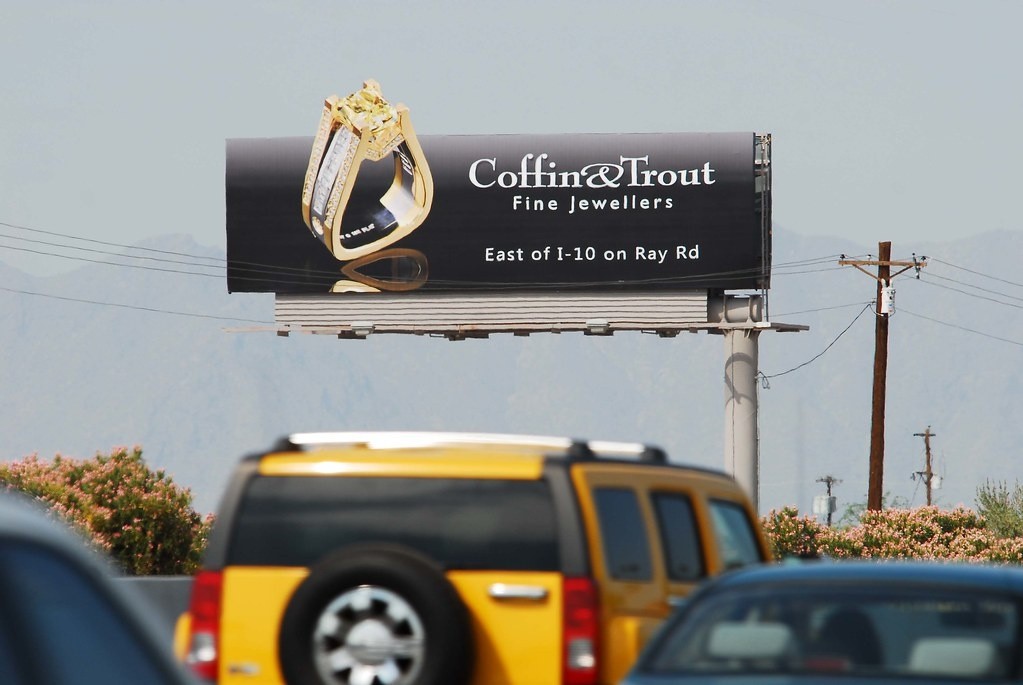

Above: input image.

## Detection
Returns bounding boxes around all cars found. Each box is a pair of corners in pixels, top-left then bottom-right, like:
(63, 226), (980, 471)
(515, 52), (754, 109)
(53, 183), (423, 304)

(0, 492), (204, 685)
(616, 559), (1023, 685)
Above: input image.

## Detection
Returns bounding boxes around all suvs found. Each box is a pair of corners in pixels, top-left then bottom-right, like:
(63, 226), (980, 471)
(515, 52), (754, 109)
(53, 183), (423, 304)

(173, 432), (771, 685)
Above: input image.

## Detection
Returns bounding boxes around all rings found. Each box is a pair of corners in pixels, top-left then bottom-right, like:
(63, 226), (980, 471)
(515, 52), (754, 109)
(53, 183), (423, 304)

(302, 80), (433, 261)
